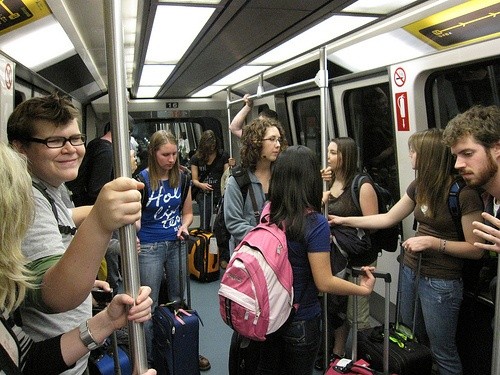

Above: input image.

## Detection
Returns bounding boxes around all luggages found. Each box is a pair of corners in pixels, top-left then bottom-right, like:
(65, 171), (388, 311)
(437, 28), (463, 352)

(89, 289), (132, 375)
(149, 231), (200, 375)
(324, 266), (398, 375)
(187, 187), (221, 283)
(357, 241), (434, 375)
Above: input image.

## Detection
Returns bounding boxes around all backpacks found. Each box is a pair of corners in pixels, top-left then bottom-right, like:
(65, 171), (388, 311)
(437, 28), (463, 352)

(328, 228), (352, 326)
(351, 173), (399, 253)
(212, 166), (249, 262)
(218, 202), (318, 342)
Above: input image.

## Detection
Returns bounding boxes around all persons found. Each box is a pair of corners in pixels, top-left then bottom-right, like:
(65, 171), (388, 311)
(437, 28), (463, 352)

(327, 128), (485, 375)
(59, 114), (150, 295)
(134, 130), (211, 370)
(442, 105), (500, 375)
(177, 132), (196, 170)
(314, 137), (378, 371)
(7, 92), (145, 375)
(230, 94), (278, 138)
(190, 131), (230, 269)
(257, 145), (376, 375)
(224, 116), (288, 375)
(0, 141), (157, 375)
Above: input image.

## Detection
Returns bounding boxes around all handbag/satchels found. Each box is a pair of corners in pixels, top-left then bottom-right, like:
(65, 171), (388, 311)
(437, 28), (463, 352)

(191, 184), (200, 199)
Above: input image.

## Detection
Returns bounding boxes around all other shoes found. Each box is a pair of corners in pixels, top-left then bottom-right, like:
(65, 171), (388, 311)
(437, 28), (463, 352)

(198, 355), (211, 371)
(220, 261), (227, 269)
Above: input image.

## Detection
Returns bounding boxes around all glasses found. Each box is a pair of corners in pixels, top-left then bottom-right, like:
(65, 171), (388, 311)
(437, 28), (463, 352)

(27, 134), (87, 148)
(264, 137), (282, 143)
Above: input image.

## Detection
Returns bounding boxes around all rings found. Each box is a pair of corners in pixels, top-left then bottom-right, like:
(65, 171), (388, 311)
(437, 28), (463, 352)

(404, 246), (408, 250)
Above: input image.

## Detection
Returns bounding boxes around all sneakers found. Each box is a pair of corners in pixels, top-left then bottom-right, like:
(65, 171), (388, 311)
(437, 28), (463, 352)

(315, 351), (345, 370)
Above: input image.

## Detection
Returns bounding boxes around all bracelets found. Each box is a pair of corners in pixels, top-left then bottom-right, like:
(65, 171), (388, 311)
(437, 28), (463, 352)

(79, 319), (107, 351)
(439, 239), (442, 253)
(442, 240), (446, 253)
